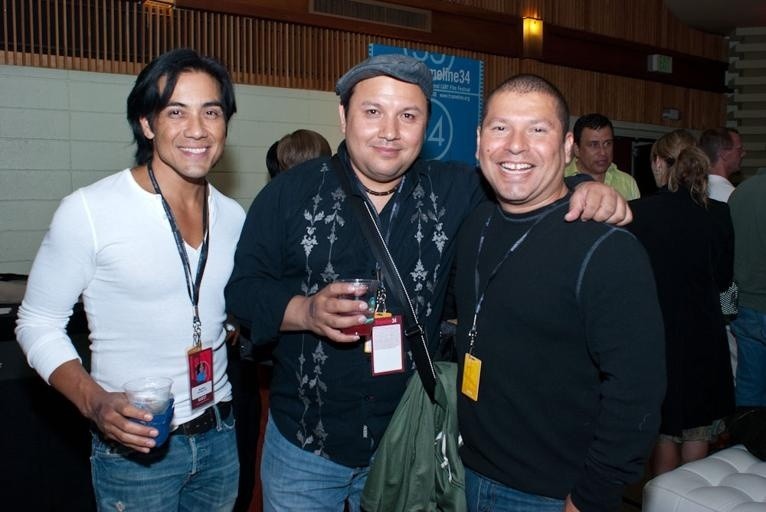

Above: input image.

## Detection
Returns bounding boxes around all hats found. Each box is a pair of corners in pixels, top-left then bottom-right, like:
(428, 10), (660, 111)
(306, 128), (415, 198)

(335, 53), (433, 99)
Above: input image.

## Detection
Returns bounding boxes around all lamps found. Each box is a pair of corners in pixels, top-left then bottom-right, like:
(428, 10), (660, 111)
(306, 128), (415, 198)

(522, 16), (544, 61)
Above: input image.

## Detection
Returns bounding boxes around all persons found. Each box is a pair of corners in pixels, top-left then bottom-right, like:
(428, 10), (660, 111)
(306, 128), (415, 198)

(450, 75), (668, 512)
(627, 130), (735, 474)
(13, 47), (248, 511)
(726, 168), (766, 454)
(699, 125), (746, 202)
(266, 129), (332, 177)
(563, 113), (641, 200)
(223, 54), (633, 512)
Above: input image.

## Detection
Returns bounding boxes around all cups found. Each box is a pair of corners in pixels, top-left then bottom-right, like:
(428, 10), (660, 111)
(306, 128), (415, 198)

(122, 377), (172, 450)
(335, 277), (376, 339)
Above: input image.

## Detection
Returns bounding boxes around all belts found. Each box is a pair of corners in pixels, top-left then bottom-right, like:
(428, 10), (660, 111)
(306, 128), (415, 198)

(169, 402), (232, 435)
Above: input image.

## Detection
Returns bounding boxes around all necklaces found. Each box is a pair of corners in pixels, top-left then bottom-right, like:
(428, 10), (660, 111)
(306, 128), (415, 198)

(359, 181), (401, 195)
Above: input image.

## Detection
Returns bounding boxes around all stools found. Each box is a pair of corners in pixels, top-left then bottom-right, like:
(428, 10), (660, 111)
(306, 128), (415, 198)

(641, 444), (766, 512)
